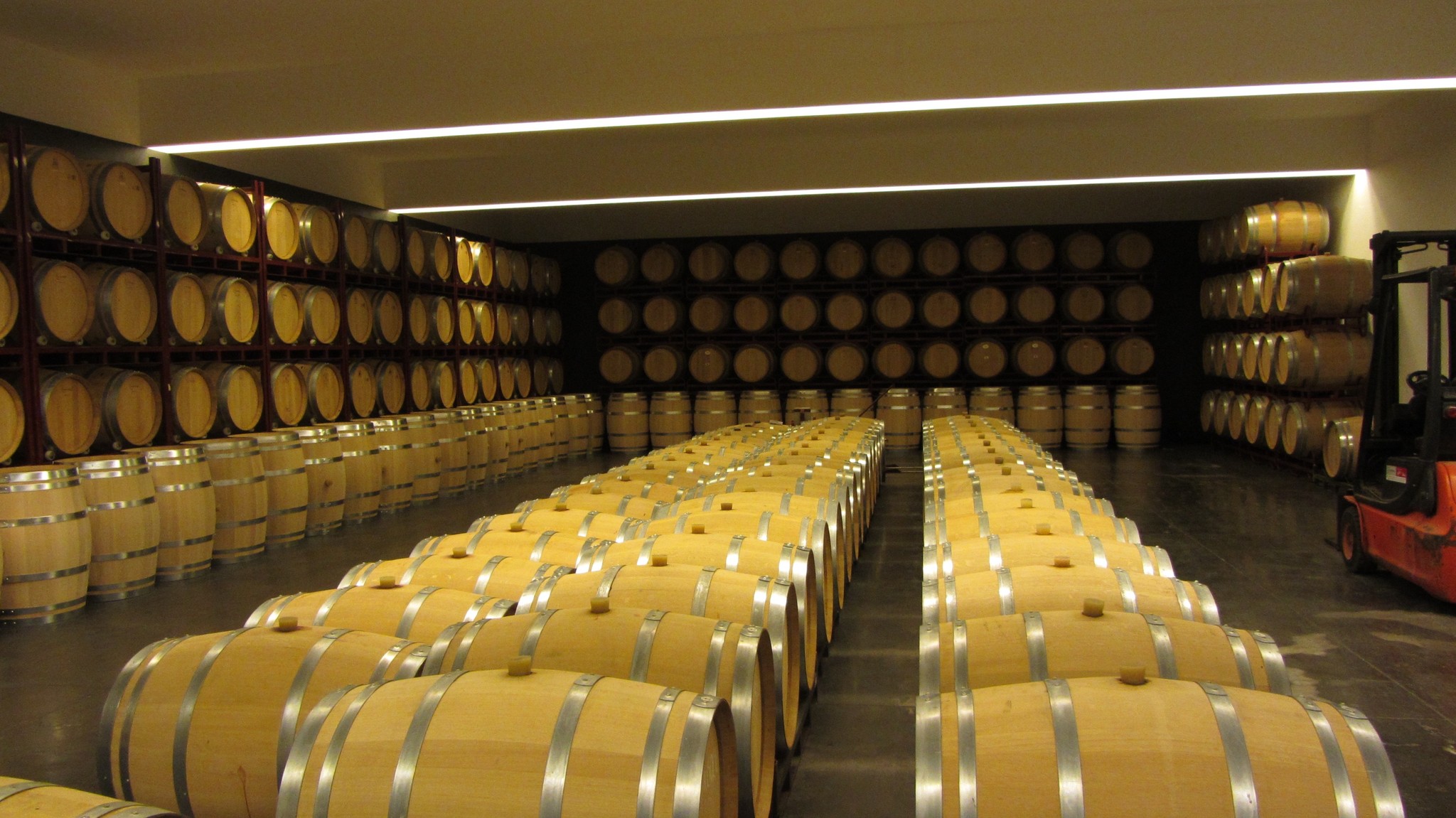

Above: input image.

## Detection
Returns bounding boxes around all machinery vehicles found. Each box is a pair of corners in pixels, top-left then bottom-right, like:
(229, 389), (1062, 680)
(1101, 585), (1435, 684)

(1310, 230), (1456, 608)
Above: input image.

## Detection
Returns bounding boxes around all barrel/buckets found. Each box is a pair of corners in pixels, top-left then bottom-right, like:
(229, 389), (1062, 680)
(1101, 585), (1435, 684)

(0, 140), (1404, 816)
